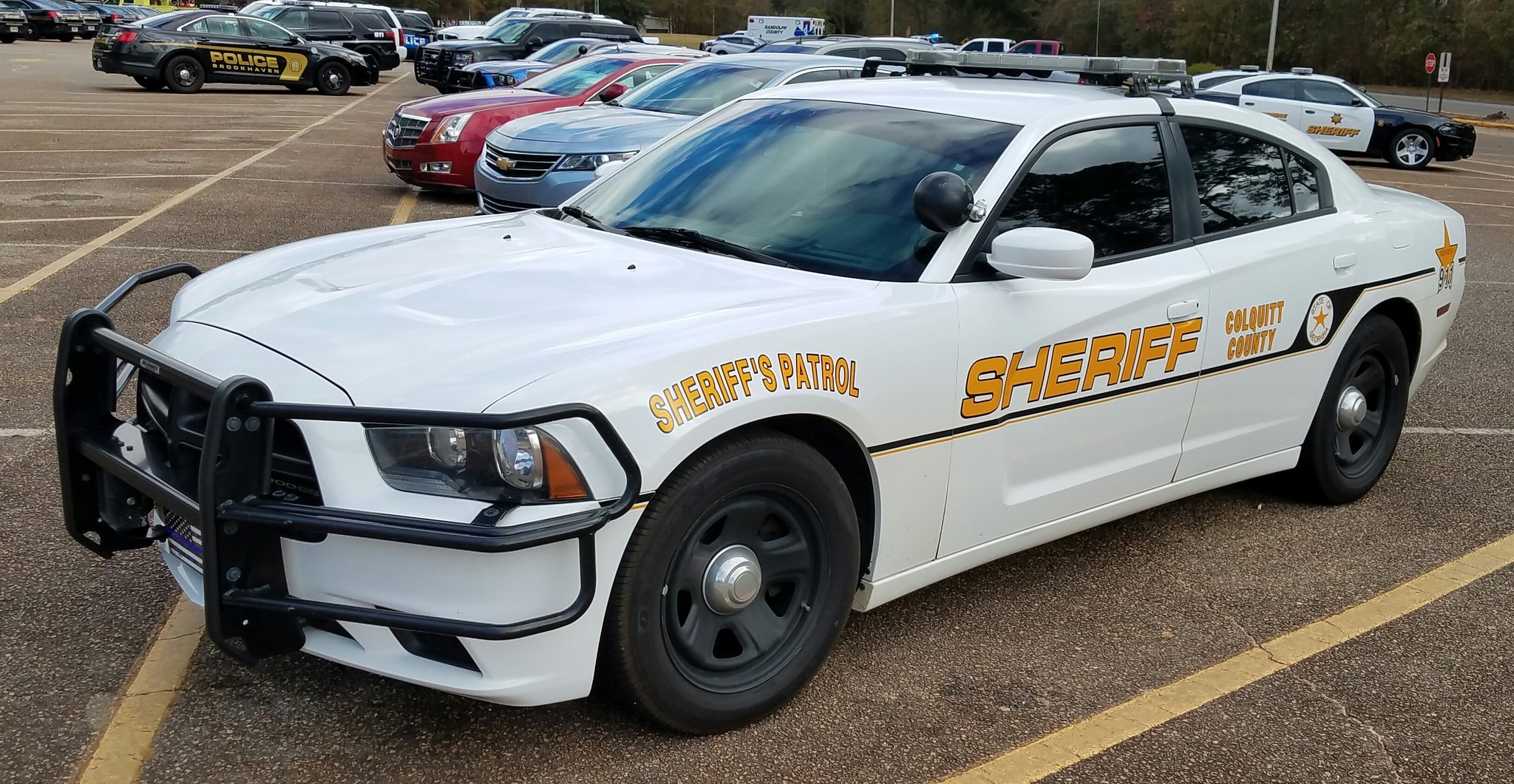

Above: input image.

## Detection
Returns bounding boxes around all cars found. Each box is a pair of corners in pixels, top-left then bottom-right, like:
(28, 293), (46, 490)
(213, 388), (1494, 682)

(47, 45), (1472, 735)
(0, 0), (201, 43)
(379, 49), (707, 200)
(472, 51), (899, 217)
(241, 0), (1091, 94)
(1148, 65), (1478, 172)
(92, 4), (381, 97)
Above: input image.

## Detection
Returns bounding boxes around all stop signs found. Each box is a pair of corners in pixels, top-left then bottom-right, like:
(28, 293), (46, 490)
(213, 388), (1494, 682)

(1424, 53), (1436, 74)
